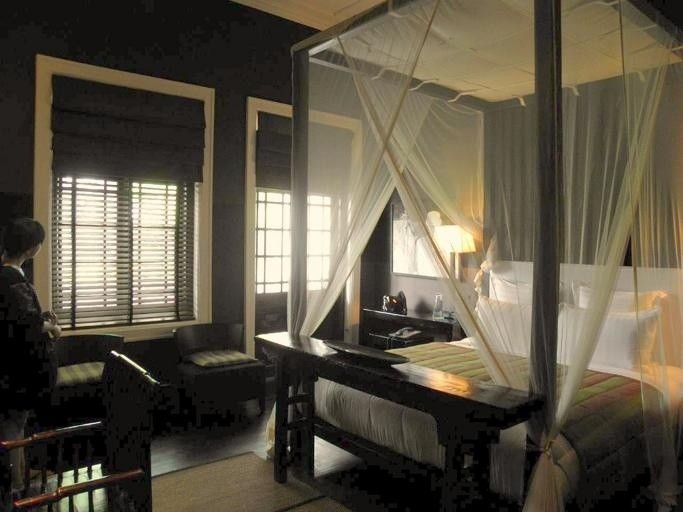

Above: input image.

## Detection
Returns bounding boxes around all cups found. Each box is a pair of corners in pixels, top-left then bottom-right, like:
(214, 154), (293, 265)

(433, 295), (444, 320)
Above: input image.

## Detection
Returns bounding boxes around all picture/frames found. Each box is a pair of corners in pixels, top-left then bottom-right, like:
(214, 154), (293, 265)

(389, 201), (462, 283)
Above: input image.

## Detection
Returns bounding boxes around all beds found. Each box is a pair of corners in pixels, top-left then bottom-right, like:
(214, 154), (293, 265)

(285, 1), (681, 511)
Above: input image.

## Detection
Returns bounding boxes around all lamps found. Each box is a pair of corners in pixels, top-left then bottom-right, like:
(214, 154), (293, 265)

(429, 224), (476, 318)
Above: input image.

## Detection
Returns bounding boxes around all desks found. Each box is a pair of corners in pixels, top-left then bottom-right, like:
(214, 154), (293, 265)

(361, 308), (465, 347)
(256, 333), (543, 512)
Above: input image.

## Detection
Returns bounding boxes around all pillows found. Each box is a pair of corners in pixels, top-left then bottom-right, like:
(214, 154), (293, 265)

(557, 302), (662, 373)
(474, 294), (544, 357)
(571, 281), (667, 313)
(486, 268), (547, 313)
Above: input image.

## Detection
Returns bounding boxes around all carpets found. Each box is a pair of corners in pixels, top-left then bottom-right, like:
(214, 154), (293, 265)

(151, 450), (350, 512)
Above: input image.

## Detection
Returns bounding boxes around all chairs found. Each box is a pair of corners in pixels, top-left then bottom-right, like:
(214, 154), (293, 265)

(54, 335), (132, 426)
(0, 350), (160, 512)
(168, 324), (269, 432)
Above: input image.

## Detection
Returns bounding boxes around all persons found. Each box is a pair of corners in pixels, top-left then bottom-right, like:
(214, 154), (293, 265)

(0, 217), (61, 502)
